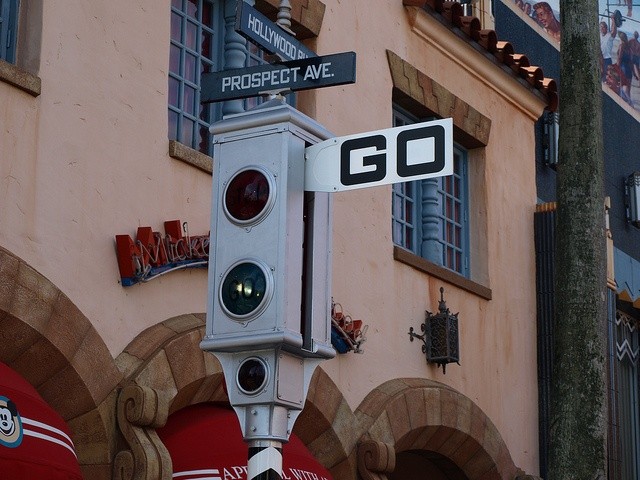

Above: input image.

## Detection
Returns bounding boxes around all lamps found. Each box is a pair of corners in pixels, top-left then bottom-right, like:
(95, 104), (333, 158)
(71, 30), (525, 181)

(543, 110), (560, 171)
(408, 286), (461, 374)
(623, 168), (640, 229)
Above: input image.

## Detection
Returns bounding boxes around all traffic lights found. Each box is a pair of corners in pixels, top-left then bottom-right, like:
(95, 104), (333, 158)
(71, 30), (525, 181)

(214, 127), (285, 405)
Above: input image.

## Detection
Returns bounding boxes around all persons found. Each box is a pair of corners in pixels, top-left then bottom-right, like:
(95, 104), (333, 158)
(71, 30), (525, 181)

(515, 0), (523, 11)
(533, 2), (560, 48)
(628, 31), (640, 82)
(617, 32), (632, 97)
(523, 2), (531, 16)
(600, 14), (617, 83)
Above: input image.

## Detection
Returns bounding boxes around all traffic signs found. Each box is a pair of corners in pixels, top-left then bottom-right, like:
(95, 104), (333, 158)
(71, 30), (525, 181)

(236, 0), (315, 60)
(202, 53), (353, 102)
